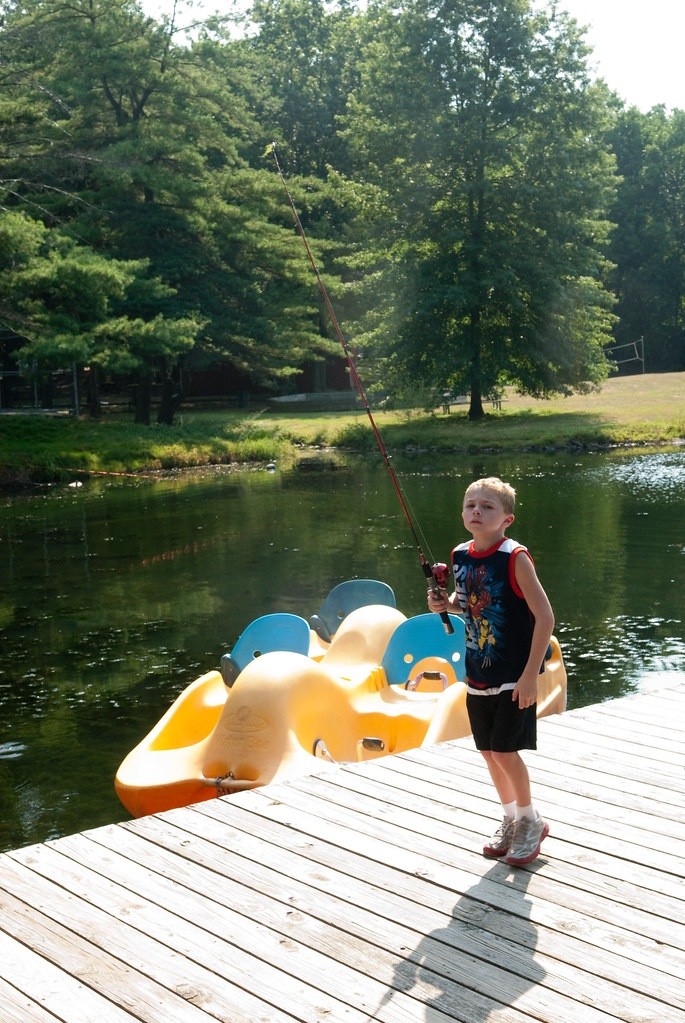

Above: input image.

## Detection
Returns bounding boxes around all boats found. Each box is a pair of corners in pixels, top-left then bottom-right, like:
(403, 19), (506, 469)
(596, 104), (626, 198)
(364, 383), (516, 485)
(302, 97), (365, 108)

(111, 584), (571, 824)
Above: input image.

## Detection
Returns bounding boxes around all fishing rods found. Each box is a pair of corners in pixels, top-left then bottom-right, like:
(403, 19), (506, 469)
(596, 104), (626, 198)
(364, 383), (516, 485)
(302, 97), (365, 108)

(270, 150), (455, 638)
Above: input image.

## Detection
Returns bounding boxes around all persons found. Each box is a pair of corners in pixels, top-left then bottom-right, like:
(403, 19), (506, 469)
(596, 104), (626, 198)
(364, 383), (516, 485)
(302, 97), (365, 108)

(429, 477), (555, 866)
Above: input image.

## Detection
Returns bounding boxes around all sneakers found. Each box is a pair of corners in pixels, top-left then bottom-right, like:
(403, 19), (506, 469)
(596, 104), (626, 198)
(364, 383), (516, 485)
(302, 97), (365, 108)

(483, 809), (550, 864)
